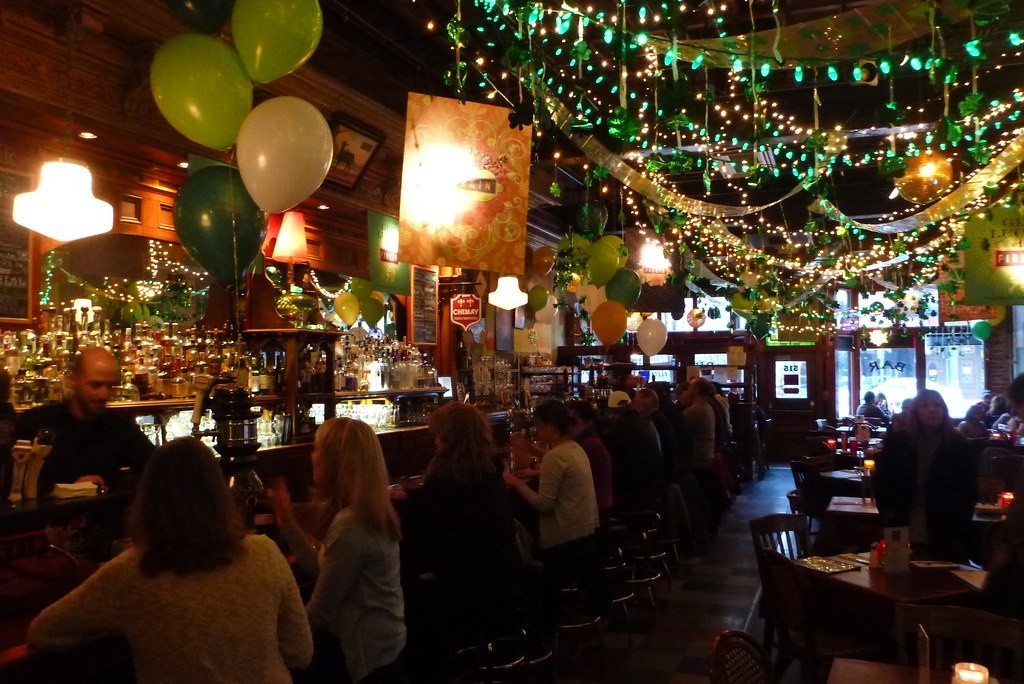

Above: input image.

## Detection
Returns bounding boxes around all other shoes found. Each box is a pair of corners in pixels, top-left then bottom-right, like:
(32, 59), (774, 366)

(756, 468), (765, 481)
(699, 528), (720, 540)
(740, 473), (753, 481)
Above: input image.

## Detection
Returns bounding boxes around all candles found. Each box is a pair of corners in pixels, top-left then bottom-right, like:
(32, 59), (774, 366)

(997, 491), (1014, 510)
(826, 439), (836, 452)
(864, 460), (875, 469)
(951, 662), (990, 684)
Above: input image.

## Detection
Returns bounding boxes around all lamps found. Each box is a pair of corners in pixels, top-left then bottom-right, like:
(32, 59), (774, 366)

(272, 212), (309, 285)
(7, 0), (116, 244)
(892, 144), (952, 205)
(488, 276), (529, 311)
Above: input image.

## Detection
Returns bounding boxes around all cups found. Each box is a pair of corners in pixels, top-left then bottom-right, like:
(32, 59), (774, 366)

(850, 440), (857, 456)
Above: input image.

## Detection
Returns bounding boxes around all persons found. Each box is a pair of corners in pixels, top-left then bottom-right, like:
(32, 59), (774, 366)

(16, 348), (158, 491)
(268, 418), (409, 683)
(390, 403), (530, 684)
(504, 376), (734, 623)
(856, 392), (890, 420)
(872, 372), (1024, 620)
(27, 434), (315, 684)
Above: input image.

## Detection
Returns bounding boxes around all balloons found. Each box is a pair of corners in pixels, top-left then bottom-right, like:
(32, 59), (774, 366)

(529, 286), (558, 323)
(231, 0), (323, 84)
(533, 247), (556, 275)
(605, 269), (641, 309)
(972, 306), (1007, 342)
(731, 293), (779, 337)
(585, 235), (629, 290)
(333, 277), (386, 332)
(172, 164), (264, 299)
(592, 301), (627, 345)
(149, 33), (254, 152)
(637, 320), (668, 356)
(237, 96), (335, 214)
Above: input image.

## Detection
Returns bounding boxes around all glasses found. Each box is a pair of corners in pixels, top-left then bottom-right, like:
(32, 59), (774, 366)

(902, 407), (911, 411)
(568, 415), (584, 428)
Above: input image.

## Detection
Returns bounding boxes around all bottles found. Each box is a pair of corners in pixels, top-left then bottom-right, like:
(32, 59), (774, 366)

(870, 542), (880, 567)
(0, 304), (284, 403)
(301, 323), (439, 394)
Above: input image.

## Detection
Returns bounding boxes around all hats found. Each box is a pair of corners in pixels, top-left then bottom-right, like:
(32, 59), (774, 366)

(608, 390), (632, 408)
(983, 389), (992, 397)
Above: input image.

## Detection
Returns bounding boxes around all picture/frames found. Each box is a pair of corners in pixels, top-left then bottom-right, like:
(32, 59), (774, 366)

(514, 305), (526, 330)
(321, 111), (385, 192)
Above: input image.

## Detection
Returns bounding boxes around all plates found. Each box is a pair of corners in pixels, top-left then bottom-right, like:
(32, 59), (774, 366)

(977, 503), (999, 512)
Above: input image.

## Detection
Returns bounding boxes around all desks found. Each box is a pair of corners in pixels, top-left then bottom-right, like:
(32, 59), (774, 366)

(825, 496), (1014, 599)
(819, 468), (873, 499)
(827, 657), (1024, 684)
(834, 449), (866, 468)
(792, 551), (988, 662)
(836, 436), (882, 445)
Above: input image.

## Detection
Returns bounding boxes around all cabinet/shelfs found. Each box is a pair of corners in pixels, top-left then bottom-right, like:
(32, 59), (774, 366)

(558, 341), (758, 406)
(459, 351), (576, 413)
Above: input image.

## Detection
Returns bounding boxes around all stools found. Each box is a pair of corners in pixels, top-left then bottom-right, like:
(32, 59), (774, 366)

(293, 418), (773, 684)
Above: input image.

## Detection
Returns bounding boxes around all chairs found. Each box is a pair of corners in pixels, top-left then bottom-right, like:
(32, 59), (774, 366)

(747, 417), (1024, 684)
